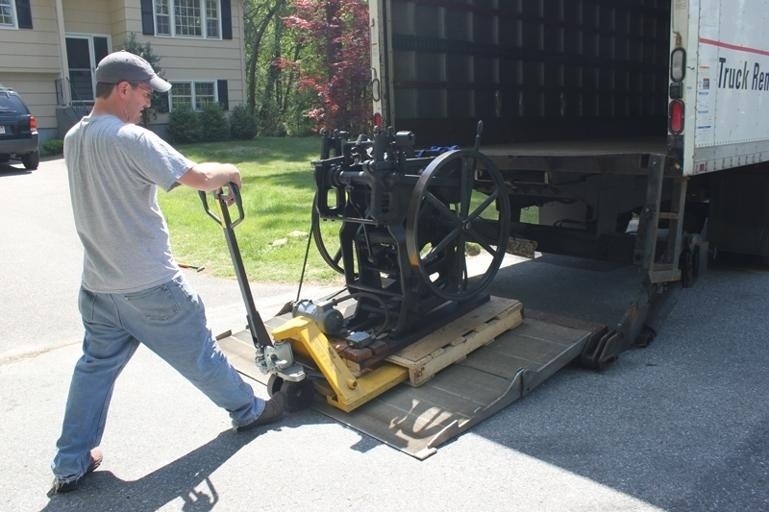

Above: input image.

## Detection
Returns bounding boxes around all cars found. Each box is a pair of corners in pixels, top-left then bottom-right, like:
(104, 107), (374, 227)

(0, 86), (40, 169)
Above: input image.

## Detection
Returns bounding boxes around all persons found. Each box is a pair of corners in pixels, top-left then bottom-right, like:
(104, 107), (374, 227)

(49, 47), (287, 493)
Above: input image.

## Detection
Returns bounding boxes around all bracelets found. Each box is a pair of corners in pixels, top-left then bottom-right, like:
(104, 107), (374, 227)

(222, 163), (231, 183)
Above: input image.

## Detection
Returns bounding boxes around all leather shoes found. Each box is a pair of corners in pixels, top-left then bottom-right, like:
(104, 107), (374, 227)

(54, 448), (102, 493)
(233, 391), (288, 432)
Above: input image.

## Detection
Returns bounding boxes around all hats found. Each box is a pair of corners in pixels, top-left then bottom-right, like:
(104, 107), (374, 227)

(95, 49), (173, 93)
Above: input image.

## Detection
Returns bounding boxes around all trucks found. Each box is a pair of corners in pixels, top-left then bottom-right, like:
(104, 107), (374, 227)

(368, 1), (768, 265)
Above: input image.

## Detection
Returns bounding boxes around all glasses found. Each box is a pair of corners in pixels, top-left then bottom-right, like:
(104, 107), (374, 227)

(132, 84), (153, 96)
